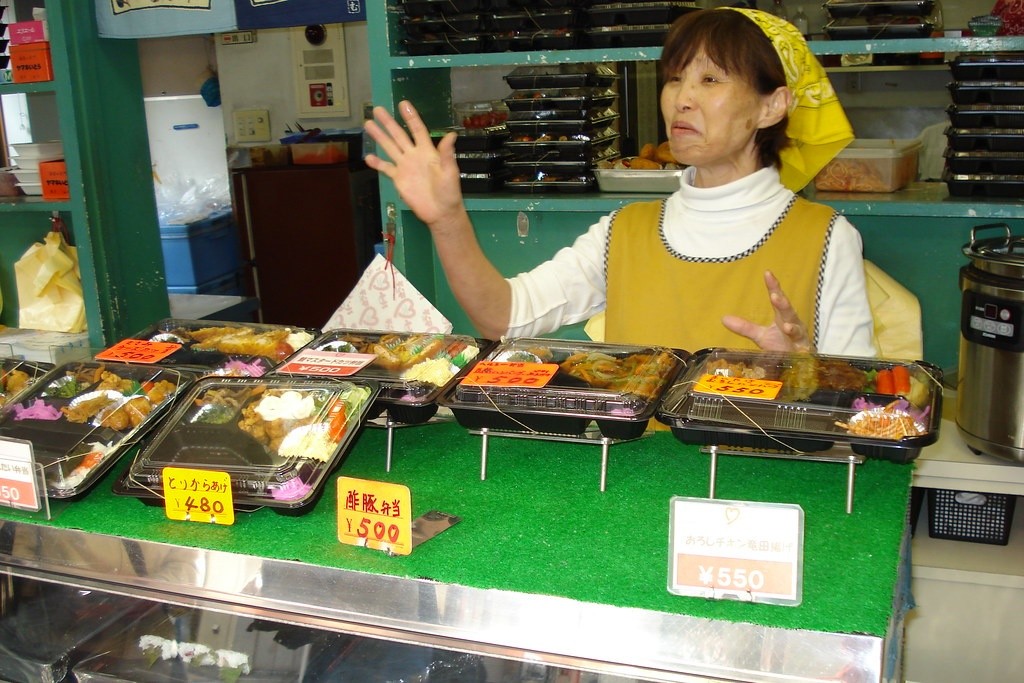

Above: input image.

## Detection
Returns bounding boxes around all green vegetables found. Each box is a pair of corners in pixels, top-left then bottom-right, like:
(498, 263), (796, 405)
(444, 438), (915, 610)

(195, 406), (236, 425)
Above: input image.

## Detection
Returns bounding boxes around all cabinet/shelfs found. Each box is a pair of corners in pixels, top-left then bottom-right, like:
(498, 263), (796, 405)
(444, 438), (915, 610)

(0, 0), (1024, 542)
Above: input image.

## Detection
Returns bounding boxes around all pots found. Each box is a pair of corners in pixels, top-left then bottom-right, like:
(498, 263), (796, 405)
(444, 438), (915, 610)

(958, 222), (1024, 463)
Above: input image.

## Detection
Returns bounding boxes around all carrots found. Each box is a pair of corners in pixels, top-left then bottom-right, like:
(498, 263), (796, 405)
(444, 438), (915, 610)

(876, 365), (911, 396)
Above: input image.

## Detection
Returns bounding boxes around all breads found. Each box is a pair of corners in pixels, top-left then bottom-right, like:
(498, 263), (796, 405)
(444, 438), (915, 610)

(629, 140), (679, 171)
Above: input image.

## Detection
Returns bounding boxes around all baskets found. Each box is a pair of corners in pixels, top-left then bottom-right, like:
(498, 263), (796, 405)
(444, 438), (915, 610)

(927, 485), (1016, 546)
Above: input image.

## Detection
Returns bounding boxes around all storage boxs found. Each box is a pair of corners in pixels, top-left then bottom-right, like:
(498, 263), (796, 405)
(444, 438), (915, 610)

(158, 204), (247, 297)
(926, 487), (1017, 546)
(38, 160), (71, 200)
(6, 21), (53, 81)
(291, 141), (347, 164)
(814, 139), (924, 192)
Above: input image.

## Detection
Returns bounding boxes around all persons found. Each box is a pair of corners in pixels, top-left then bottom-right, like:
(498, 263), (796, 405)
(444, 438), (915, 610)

(364, 6), (873, 356)
